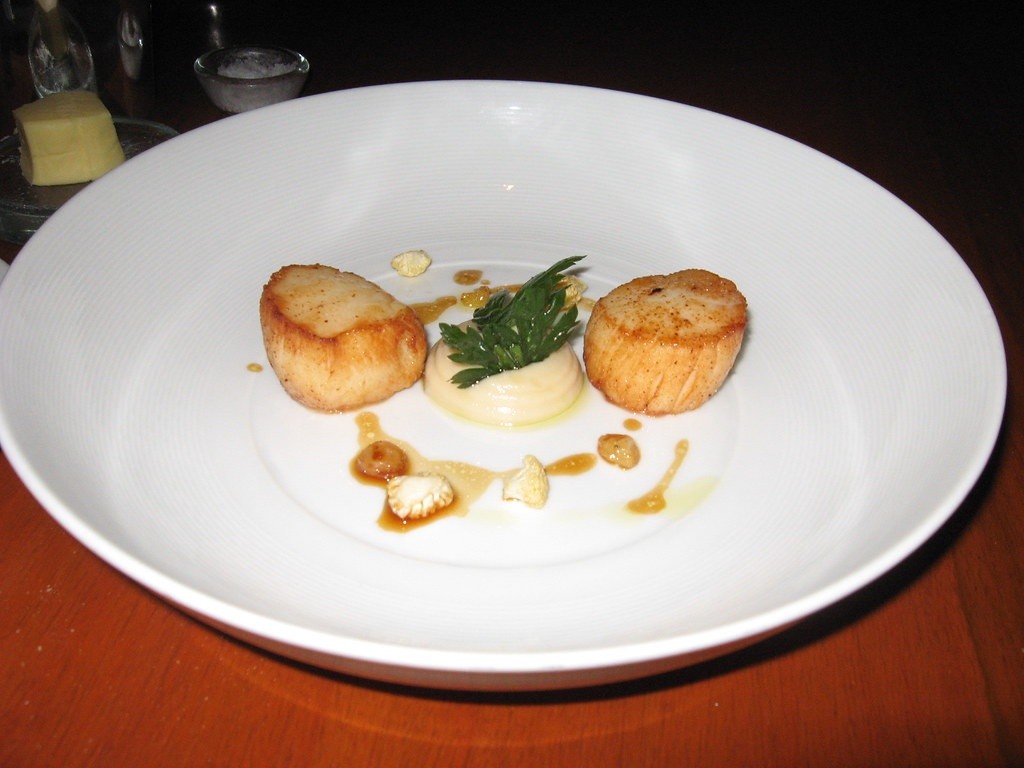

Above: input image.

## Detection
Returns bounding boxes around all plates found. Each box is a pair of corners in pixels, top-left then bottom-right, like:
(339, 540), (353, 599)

(0, 78), (1007, 692)
(1, 115), (179, 247)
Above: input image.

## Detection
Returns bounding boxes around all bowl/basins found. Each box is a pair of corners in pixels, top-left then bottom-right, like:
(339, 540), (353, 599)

(193, 44), (310, 113)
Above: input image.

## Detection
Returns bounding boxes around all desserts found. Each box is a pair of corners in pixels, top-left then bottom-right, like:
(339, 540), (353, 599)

(14, 88), (125, 186)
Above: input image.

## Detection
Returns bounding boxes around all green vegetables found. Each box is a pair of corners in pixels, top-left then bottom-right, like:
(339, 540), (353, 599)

(449, 255), (587, 389)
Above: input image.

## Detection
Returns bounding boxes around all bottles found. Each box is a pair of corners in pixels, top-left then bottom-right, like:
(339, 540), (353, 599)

(27, 1), (90, 97)
